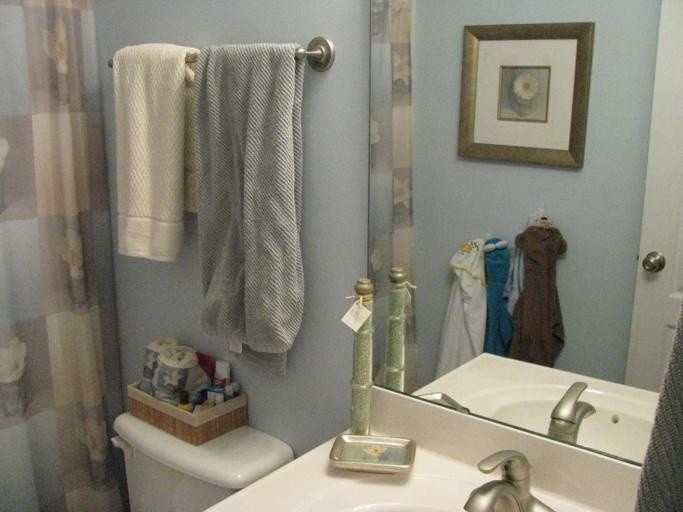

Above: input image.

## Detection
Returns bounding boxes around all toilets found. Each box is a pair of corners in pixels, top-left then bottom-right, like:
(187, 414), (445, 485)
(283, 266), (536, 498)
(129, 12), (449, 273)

(112, 411), (295, 511)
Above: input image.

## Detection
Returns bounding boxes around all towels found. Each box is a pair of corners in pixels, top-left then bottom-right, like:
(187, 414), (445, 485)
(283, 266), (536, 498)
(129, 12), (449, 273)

(512, 225), (567, 368)
(435, 239), (486, 378)
(110, 42), (201, 265)
(191, 41), (306, 377)
(483, 238), (513, 357)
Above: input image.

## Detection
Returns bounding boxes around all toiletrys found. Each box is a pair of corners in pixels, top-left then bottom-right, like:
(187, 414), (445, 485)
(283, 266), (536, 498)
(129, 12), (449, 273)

(214, 360), (230, 388)
(177, 360), (241, 415)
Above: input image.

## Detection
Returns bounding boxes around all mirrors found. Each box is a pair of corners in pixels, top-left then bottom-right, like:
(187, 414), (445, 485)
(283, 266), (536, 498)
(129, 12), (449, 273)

(369, 0), (683, 471)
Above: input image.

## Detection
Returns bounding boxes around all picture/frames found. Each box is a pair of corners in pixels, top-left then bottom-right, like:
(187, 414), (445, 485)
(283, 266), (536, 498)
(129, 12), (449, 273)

(457, 21), (594, 169)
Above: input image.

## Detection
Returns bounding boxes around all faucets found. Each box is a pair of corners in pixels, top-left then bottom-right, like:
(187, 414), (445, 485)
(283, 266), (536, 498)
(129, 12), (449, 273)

(549, 381), (597, 446)
(463, 452), (533, 511)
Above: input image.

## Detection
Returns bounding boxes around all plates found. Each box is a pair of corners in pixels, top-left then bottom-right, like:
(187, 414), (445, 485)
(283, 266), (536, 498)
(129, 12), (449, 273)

(329, 434), (417, 474)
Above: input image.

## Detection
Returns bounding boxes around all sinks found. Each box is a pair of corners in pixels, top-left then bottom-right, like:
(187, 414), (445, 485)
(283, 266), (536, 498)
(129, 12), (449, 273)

(490, 399), (654, 460)
